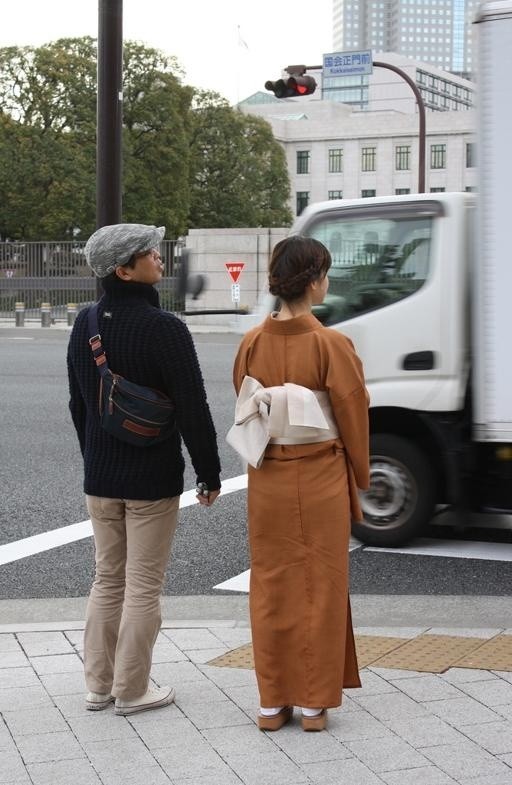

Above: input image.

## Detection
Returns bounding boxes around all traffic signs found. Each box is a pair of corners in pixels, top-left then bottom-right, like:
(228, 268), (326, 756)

(323, 50), (374, 78)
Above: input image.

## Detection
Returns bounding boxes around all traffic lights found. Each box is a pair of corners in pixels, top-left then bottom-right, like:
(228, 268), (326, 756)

(265, 75), (317, 98)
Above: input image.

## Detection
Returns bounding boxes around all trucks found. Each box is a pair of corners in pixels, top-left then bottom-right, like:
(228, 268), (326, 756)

(249, 2), (511, 546)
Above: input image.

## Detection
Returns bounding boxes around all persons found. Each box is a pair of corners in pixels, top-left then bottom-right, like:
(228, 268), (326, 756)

(231, 235), (370, 732)
(65, 223), (220, 715)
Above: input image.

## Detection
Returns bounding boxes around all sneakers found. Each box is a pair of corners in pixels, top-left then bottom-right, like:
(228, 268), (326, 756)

(113, 685), (176, 716)
(84, 690), (113, 711)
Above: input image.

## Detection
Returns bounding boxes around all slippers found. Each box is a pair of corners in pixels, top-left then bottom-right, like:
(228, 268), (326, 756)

(256, 705), (293, 732)
(302, 708), (329, 732)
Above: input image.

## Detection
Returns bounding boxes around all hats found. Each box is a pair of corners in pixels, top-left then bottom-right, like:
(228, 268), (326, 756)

(83, 223), (166, 280)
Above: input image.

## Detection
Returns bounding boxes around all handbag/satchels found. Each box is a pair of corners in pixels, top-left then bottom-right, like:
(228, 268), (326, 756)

(97, 369), (179, 449)
